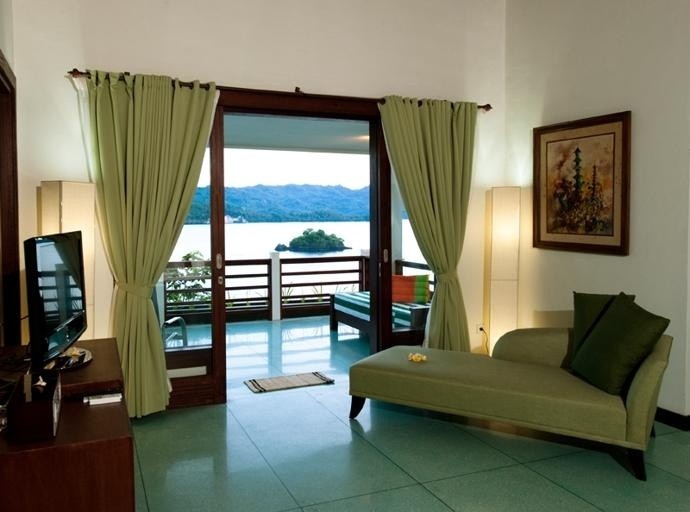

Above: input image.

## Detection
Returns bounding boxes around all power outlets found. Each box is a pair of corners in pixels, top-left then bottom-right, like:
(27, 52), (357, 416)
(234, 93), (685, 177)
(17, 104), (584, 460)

(477, 323), (484, 336)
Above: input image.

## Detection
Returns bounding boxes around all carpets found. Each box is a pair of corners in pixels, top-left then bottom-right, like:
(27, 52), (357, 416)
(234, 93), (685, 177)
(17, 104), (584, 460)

(244, 370), (335, 393)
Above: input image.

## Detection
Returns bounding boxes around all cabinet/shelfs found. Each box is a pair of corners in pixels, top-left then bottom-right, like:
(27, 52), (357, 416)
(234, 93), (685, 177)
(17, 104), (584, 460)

(0, 335), (136, 512)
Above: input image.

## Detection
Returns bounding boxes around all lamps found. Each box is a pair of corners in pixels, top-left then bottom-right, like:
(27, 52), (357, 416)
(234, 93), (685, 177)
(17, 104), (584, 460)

(485, 185), (521, 357)
(36, 180), (94, 339)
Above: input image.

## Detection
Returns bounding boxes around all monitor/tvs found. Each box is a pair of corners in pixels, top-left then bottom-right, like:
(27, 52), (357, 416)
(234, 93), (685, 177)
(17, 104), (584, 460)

(24, 230), (93, 376)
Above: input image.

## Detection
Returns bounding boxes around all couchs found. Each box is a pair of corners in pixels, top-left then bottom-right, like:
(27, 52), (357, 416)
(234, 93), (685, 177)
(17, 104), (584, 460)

(329, 259), (438, 347)
(350, 327), (674, 482)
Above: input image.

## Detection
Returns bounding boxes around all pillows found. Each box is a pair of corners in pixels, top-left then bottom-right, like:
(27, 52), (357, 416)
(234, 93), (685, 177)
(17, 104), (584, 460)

(391, 274), (428, 303)
(567, 289), (670, 396)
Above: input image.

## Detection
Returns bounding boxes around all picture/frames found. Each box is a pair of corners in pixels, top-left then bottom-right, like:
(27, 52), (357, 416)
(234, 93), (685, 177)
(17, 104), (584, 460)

(532, 109), (628, 256)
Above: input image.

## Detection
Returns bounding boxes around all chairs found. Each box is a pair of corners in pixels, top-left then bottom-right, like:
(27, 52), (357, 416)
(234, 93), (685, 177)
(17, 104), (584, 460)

(160, 316), (187, 349)
